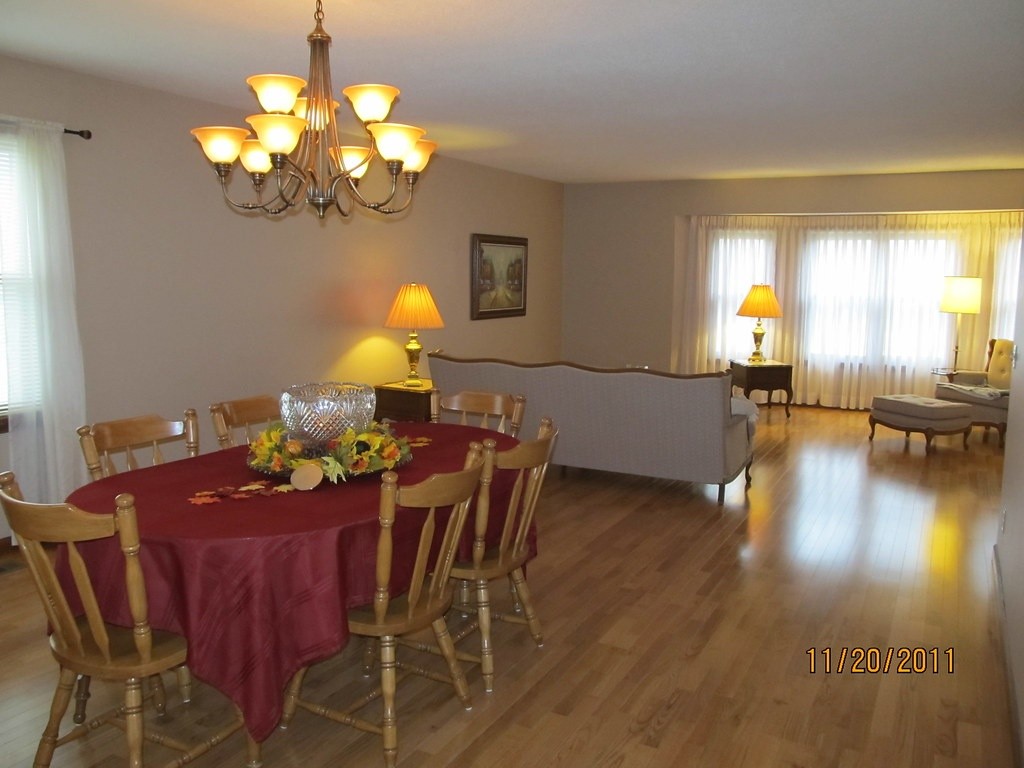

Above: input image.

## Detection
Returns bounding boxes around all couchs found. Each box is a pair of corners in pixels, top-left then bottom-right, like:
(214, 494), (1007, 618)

(935, 337), (1014, 449)
(427, 346), (760, 507)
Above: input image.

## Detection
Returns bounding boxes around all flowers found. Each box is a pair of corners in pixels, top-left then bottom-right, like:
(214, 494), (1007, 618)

(247, 425), (412, 482)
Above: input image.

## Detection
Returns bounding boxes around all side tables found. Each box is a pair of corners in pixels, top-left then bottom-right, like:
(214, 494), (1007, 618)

(728, 359), (794, 418)
(374, 381), (431, 425)
(930, 369), (958, 384)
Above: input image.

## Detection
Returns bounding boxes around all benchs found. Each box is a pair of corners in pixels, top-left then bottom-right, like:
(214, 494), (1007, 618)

(868, 393), (974, 453)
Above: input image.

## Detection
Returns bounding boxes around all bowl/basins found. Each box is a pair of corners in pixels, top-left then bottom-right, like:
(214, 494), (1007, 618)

(280, 382), (376, 441)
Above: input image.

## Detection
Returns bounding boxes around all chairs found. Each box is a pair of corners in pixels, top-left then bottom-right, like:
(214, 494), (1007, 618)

(394, 415), (560, 694)
(75, 405), (199, 487)
(430, 386), (527, 441)
(274, 443), (488, 767)
(0, 468), (264, 768)
(206, 396), (283, 450)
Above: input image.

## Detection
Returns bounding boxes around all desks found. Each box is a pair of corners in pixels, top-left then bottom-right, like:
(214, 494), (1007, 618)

(48, 418), (538, 767)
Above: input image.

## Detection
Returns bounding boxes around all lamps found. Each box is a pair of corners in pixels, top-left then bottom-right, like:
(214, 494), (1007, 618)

(386, 281), (447, 389)
(937, 275), (982, 371)
(188, 1), (438, 225)
(735, 282), (785, 366)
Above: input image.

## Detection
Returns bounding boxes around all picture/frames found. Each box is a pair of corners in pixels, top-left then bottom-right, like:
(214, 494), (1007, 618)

(471, 233), (529, 321)
